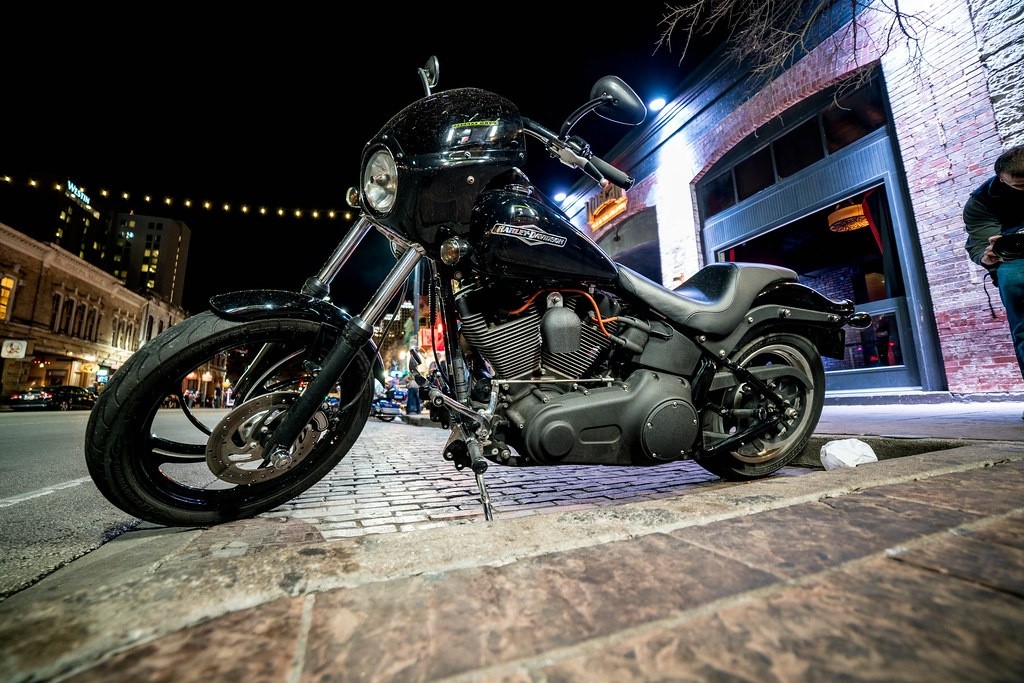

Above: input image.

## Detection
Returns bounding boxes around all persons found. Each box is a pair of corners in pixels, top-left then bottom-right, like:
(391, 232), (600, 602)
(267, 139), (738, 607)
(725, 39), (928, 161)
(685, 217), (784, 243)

(390, 374), (410, 389)
(86, 382), (221, 408)
(963, 144), (1024, 421)
(429, 353), (441, 371)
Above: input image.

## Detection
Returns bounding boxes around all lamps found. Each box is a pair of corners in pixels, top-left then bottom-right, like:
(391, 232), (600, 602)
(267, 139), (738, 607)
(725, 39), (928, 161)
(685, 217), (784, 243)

(828, 199), (870, 232)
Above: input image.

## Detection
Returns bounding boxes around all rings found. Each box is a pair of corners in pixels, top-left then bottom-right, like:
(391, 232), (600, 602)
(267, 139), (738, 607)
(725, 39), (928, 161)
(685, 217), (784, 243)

(983, 250), (988, 257)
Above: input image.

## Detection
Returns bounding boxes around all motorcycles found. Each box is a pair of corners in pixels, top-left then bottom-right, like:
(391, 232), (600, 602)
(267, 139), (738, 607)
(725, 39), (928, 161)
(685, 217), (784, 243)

(82, 56), (871, 526)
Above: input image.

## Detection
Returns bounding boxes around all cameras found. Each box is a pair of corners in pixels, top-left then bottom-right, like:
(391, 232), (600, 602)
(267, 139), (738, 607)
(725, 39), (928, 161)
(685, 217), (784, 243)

(992, 225), (1024, 261)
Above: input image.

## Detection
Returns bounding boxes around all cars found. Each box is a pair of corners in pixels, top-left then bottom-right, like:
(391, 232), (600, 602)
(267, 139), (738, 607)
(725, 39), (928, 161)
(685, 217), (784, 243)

(10, 385), (99, 411)
